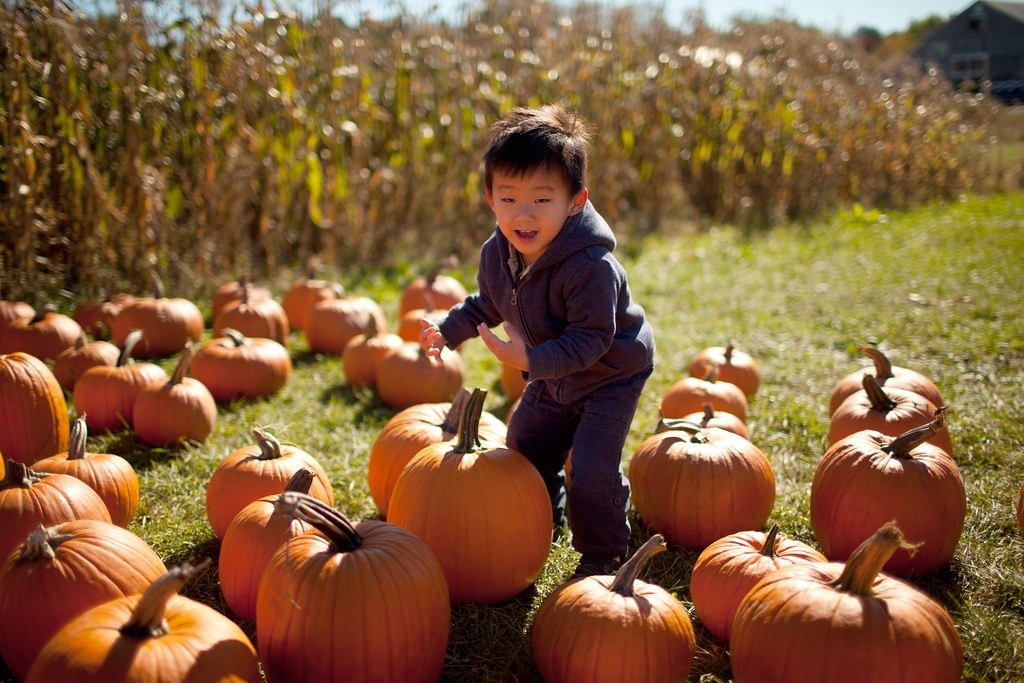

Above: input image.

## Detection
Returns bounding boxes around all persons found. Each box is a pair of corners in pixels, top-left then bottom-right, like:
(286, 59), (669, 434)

(419, 104), (655, 585)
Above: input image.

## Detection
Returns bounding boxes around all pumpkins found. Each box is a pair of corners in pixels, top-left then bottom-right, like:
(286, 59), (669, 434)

(0, 255), (968, 683)
(1016, 482), (1024, 532)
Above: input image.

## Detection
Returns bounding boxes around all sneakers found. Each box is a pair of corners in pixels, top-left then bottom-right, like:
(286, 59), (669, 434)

(568, 558), (622, 583)
(552, 526), (564, 544)
